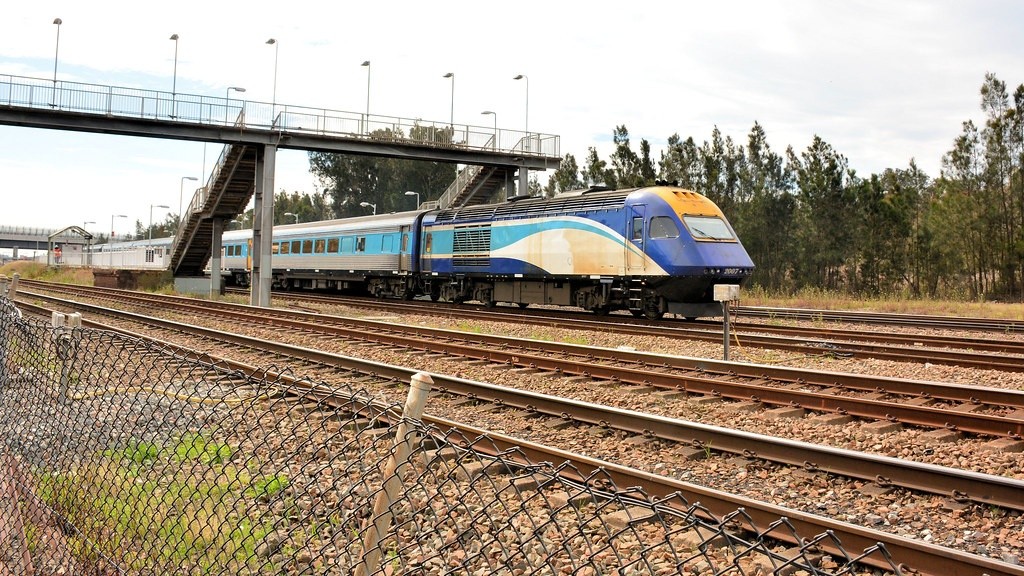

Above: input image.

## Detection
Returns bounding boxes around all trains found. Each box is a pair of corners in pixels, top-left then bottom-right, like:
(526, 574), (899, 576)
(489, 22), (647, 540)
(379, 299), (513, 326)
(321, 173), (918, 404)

(83, 180), (757, 323)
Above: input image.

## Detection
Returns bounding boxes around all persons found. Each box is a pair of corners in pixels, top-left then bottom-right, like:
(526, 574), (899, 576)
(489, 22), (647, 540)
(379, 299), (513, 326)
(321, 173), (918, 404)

(54, 245), (62, 264)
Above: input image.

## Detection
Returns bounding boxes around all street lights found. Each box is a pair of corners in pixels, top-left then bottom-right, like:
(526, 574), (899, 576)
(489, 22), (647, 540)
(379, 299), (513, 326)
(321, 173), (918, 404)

(360, 202), (376, 215)
(149, 204), (170, 248)
(110, 215), (127, 267)
(265, 39), (279, 124)
(179, 177), (198, 227)
(84, 222), (96, 253)
(481, 111), (496, 151)
(231, 220), (243, 230)
(284, 212), (298, 224)
(52, 18), (62, 109)
(443, 72), (455, 143)
(405, 191), (419, 210)
(513, 74), (529, 151)
(361, 61), (370, 135)
(225, 86), (246, 126)
(170, 34), (179, 119)
